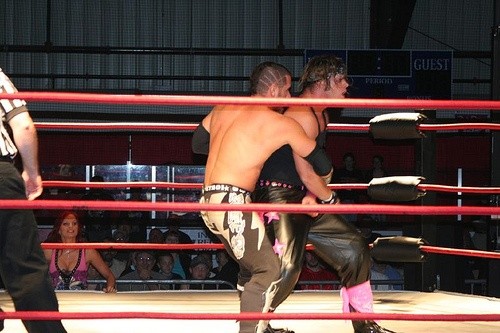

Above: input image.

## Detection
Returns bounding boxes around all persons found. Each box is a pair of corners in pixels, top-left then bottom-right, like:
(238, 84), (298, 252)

(0, 67), (67, 333)
(252, 53), (396, 333)
(192, 61), (334, 333)
(33, 176), (403, 291)
(42, 211), (118, 294)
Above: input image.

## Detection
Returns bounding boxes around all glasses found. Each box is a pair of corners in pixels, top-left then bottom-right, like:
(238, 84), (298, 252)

(136, 257), (154, 262)
(113, 237), (124, 241)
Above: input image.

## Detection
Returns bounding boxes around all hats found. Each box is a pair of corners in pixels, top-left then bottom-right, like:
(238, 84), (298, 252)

(190, 256), (209, 269)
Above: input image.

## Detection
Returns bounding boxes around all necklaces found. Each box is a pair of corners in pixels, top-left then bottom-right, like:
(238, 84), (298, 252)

(66, 249), (72, 257)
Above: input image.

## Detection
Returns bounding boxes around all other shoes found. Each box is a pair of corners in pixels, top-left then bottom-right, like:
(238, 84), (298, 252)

(352, 320), (396, 333)
(264, 323), (295, 333)
